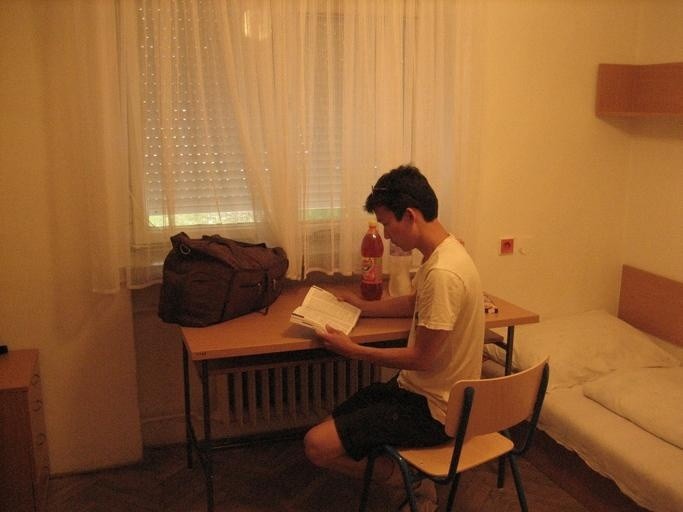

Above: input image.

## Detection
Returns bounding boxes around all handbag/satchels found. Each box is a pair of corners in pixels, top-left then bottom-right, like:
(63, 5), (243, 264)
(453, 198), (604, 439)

(158, 232), (288, 327)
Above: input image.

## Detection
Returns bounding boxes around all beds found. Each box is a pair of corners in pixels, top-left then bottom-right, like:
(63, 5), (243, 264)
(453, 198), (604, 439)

(482, 313), (682, 512)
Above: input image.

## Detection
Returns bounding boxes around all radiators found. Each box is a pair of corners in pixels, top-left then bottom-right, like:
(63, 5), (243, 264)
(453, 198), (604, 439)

(204, 348), (381, 439)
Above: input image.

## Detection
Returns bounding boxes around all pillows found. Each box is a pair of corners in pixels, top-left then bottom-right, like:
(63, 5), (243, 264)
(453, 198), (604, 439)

(489, 314), (678, 388)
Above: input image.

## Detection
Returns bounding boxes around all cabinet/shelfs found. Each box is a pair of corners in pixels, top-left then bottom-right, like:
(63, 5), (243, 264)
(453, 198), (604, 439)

(0, 348), (50, 512)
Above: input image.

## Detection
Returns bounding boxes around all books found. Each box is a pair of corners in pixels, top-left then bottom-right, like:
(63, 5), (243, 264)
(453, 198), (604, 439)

(288, 285), (361, 339)
(484, 295), (498, 314)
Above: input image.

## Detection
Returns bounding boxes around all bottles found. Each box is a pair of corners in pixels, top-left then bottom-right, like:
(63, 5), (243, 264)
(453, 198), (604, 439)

(387, 238), (412, 299)
(358, 221), (384, 301)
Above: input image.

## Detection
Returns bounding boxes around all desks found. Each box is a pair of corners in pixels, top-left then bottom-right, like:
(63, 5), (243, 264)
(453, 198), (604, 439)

(178, 277), (541, 512)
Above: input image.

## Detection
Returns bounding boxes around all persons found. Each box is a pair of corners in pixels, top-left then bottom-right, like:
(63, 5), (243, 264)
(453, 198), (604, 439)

(303, 163), (485, 511)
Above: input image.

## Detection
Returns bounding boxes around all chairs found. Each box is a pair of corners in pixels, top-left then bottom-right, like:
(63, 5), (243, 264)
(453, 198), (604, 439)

(359, 354), (551, 512)
